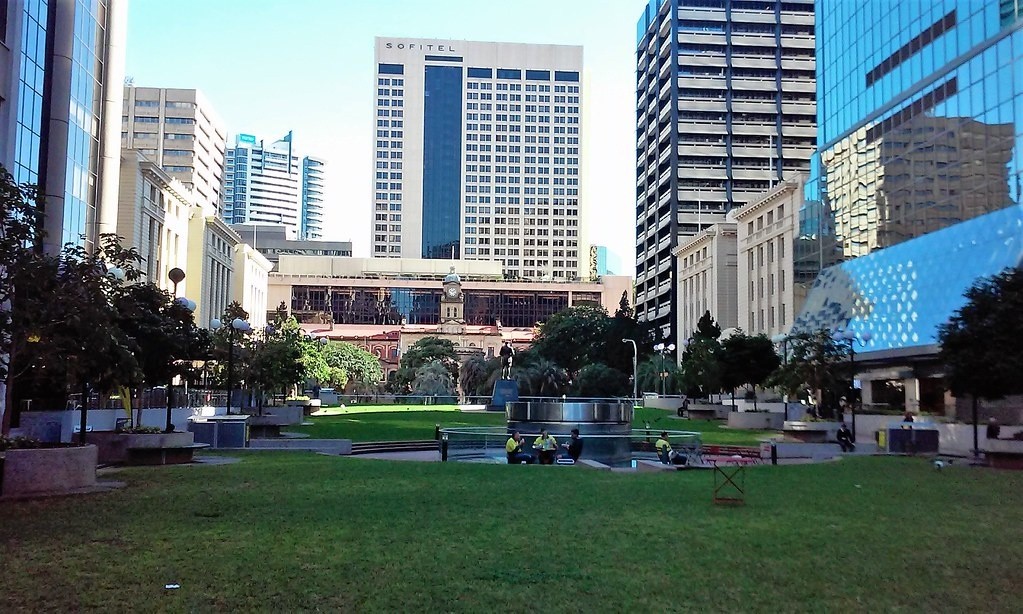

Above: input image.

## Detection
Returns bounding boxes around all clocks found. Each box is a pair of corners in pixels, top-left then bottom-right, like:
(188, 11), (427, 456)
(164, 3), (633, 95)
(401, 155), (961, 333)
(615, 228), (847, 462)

(449, 289), (456, 297)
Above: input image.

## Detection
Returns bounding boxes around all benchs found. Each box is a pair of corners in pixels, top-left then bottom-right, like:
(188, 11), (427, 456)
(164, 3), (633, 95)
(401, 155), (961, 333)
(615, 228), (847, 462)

(697, 444), (774, 465)
(984, 451), (1023, 470)
(132, 441), (211, 466)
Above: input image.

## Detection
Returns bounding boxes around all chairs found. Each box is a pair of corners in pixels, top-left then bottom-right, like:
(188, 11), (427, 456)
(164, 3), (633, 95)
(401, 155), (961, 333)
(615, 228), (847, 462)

(655, 445), (687, 465)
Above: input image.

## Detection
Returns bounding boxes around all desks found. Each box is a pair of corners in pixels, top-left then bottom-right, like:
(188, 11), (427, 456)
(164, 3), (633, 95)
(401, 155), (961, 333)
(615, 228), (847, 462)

(705, 457), (759, 508)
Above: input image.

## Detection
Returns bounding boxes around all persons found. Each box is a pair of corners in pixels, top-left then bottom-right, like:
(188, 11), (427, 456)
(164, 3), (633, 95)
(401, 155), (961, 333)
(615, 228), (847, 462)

(505, 431), (532, 464)
(901, 410), (915, 429)
(555, 429), (583, 464)
(836, 423), (856, 452)
(656, 431), (687, 464)
(312, 380), (322, 399)
(985, 416), (1000, 441)
(65, 395), (77, 411)
(531, 428), (558, 465)
(499, 342), (514, 380)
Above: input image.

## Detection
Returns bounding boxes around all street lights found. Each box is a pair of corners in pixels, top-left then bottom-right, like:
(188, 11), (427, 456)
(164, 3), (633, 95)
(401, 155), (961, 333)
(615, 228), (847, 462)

(263, 327), (275, 409)
(166, 295), (196, 433)
(683, 338), (696, 404)
(622, 338), (637, 406)
(654, 343), (675, 398)
(211, 319), (253, 415)
(832, 331), (871, 442)
(311, 334), (328, 388)
(771, 333), (793, 421)
(80, 262), (125, 445)
